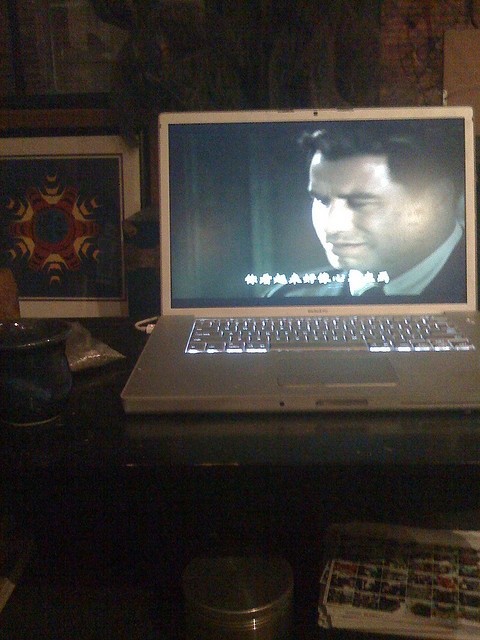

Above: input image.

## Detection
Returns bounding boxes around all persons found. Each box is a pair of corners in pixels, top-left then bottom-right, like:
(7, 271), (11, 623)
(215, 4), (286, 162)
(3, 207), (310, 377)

(267, 129), (466, 296)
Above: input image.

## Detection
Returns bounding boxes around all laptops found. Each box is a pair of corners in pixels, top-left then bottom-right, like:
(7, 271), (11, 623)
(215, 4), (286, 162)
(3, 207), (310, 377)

(120, 107), (479, 414)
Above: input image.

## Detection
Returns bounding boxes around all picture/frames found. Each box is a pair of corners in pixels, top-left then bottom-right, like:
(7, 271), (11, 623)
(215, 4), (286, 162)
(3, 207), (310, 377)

(0, 122), (150, 332)
